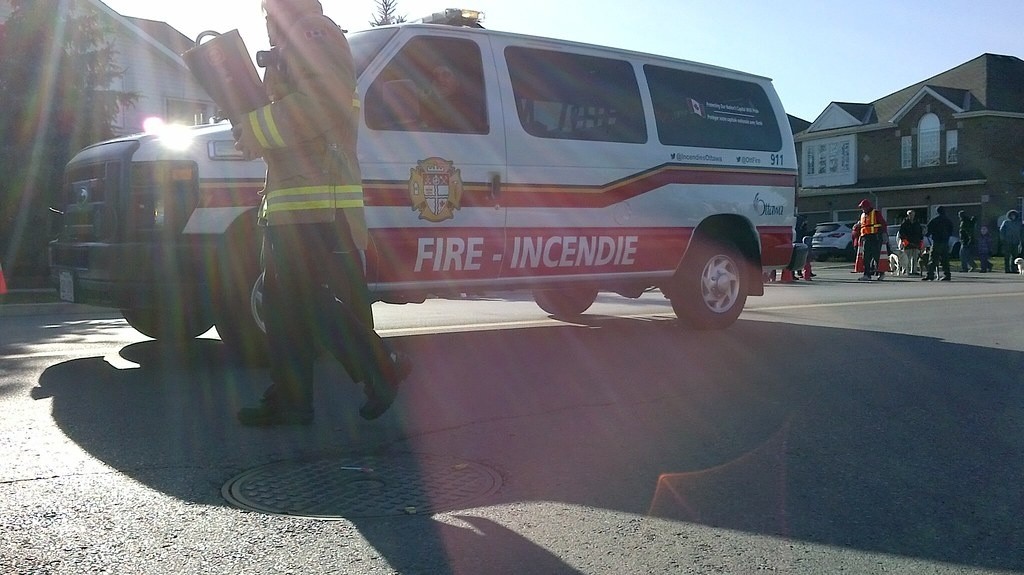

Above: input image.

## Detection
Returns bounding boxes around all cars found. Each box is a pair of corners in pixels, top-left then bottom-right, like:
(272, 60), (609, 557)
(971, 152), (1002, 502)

(885, 225), (963, 259)
(810, 220), (861, 262)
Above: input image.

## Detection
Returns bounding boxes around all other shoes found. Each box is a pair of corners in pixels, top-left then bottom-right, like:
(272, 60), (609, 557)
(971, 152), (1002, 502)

(1005, 271), (1020, 274)
(877, 272), (886, 281)
(798, 274), (804, 278)
(792, 276), (799, 280)
(940, 276), (951, 281)
(969, 265), (979, 272)
(810, 273), (817, 277)
(921, 277), (935, 281)
(958, 269), (969, 272)
(978, 268), (988, 273)
(858, 274), (871, 281)
(988, 263), (993, 273)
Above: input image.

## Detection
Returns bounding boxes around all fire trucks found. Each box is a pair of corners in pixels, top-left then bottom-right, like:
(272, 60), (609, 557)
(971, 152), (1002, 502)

(44, 8), (808, 355)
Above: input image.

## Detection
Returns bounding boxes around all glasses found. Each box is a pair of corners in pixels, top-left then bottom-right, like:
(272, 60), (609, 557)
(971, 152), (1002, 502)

(431, 70), (455, 82)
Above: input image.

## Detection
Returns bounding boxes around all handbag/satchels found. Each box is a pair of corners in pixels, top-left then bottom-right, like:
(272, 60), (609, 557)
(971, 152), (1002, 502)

(181, 29), (271, 139)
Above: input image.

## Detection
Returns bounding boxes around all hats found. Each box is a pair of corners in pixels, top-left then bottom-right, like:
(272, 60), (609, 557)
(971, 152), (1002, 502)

(434, 58), (471, 79)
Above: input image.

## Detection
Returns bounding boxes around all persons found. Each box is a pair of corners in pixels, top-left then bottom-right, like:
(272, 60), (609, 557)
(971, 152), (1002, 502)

(763, 199), (1024, 284)
(419, 60), (482, 130)
(228, 0), (414, 428)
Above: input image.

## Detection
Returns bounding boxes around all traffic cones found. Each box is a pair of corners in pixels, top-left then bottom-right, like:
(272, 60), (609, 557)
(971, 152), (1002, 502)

(776, 266), (794, 283)
(852, 236), (865, 273)
(874, 243), (894, 272)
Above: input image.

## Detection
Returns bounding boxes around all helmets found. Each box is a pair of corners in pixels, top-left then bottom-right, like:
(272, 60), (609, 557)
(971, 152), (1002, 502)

(858, 199), (873, 208)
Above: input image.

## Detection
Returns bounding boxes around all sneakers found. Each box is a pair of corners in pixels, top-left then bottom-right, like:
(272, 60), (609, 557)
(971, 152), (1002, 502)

(358, 349), (414, 420)
(236, 384), (315, 425)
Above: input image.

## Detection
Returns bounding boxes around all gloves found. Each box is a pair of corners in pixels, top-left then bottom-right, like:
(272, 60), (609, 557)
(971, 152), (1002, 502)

(919, 241), (924, 249)
(902, 240), (908, 246)
